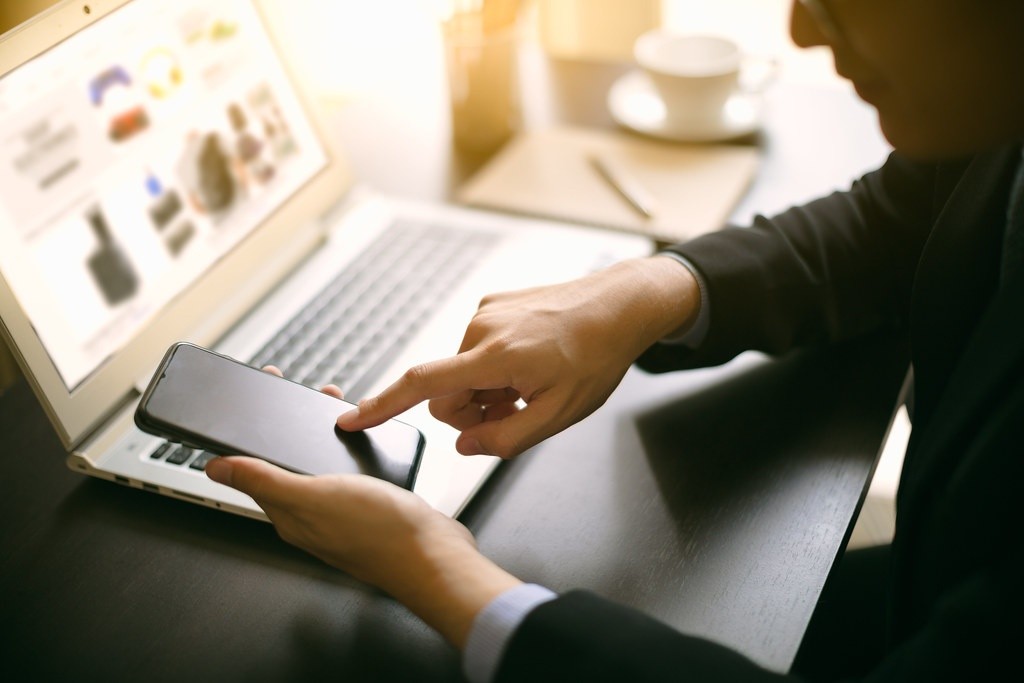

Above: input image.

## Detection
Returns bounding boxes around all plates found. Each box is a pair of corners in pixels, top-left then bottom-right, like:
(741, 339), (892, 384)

(608, 70), (775, 143)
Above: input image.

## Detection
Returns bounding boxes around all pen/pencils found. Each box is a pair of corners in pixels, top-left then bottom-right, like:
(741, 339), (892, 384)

(588, 155), (653, 219)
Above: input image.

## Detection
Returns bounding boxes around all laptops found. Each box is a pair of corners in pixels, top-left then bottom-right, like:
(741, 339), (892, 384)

(0, 1), (658, 525)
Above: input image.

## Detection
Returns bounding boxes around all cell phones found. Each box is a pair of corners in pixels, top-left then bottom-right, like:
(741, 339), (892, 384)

(133, 342), (425, 493)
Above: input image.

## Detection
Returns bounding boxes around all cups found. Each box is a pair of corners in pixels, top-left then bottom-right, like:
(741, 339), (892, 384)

(635, 31), (747, 113)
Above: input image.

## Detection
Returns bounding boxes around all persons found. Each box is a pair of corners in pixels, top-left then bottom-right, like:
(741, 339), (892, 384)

(203, 1), (1024, 683)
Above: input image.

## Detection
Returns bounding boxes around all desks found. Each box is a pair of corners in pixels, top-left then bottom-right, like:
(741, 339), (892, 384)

(0, 31), (913, 682)
